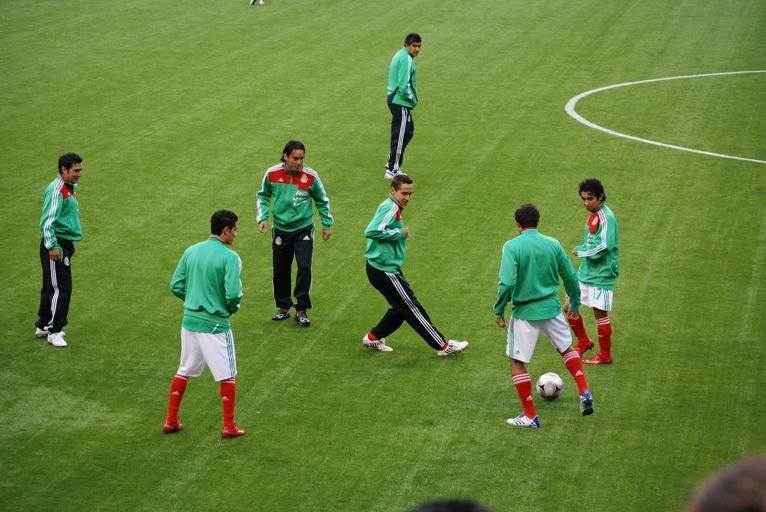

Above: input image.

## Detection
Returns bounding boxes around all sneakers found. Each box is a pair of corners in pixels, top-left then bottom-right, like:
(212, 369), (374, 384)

(220, 425), (246, 439)
(295, 309), (311, 328)
(46, 332), (68, 347)
(582, 353), (614, 365)
(272, 308), (291, 320)
(384, 169), (407, 180)
(385, 162), (390, 168)
(162, 422), (184, 434)
(437, 337), (469, 357)
(506, 412), (542, 429)
(362, 333), (393, 353)
(577, 337), (595, 358)
(34, 326), (66, 337)
(579, 390), (594, 416)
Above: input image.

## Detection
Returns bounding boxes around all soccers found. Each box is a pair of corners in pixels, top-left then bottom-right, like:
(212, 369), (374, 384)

(536, 372), (564, 398)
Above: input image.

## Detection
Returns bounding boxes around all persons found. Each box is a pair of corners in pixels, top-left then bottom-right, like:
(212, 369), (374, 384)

(563, 179), (619, 365)
(493, 203), (593, 429)
(163, 209), (246, 439)
(35, 152), (82, 346)
(384, 32), (421, 181)
(256, 139), (334, 326)
(362, 174), (470, 358)
(683, 455), (765, 510)
